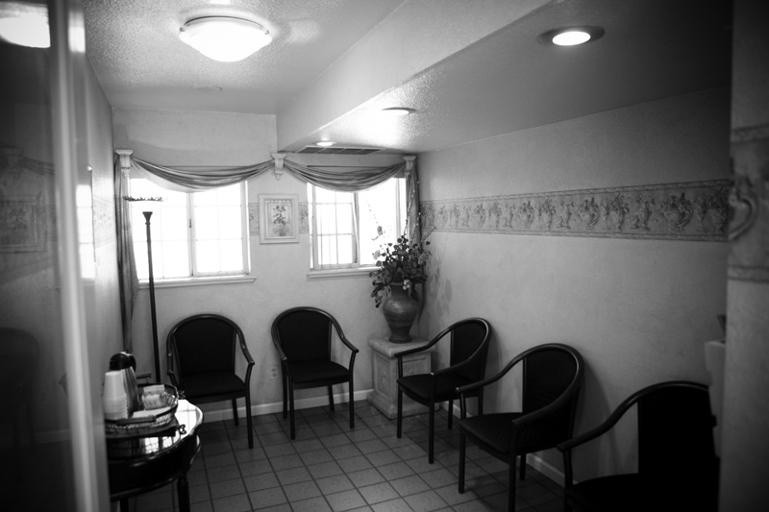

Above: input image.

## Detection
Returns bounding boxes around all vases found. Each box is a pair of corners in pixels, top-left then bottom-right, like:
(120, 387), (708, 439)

(381, 283), (418, 343)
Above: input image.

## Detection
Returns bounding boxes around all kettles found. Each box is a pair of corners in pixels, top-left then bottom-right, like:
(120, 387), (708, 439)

(108, 352), (142, 410)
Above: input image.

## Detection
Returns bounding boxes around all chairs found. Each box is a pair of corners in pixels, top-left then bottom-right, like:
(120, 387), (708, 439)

(167, 314), (255, 449)
(556, 381), (721, 512)
(0, 328), (43, 464)
(270, 306), (361, 439)
(394, 317), (491, 465)
(456, 342), (585, 512)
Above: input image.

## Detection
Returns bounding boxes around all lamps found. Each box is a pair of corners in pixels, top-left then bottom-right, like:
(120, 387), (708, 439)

(176, 15), (271, 65)
(123, 194), (159, 385)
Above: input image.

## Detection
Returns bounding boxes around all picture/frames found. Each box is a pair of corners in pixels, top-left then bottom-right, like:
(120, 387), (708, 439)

(0, 192), (48, 254)
(257, 194), (298, 245)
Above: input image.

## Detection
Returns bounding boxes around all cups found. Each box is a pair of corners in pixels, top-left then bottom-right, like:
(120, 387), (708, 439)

(103, 369), (127, 419)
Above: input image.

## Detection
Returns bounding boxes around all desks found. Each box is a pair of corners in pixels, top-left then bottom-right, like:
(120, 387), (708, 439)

(103, 398), (204, 512)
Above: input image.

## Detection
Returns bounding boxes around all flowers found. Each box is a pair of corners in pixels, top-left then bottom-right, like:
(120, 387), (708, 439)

(369, 235), (432, 308)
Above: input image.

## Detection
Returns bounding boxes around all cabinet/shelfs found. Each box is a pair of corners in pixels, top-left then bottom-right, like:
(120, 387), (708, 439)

(365, 336), (438, 420)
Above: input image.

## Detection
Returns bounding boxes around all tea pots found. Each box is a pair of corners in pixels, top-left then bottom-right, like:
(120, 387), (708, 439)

(141, 383), (168, 409)
